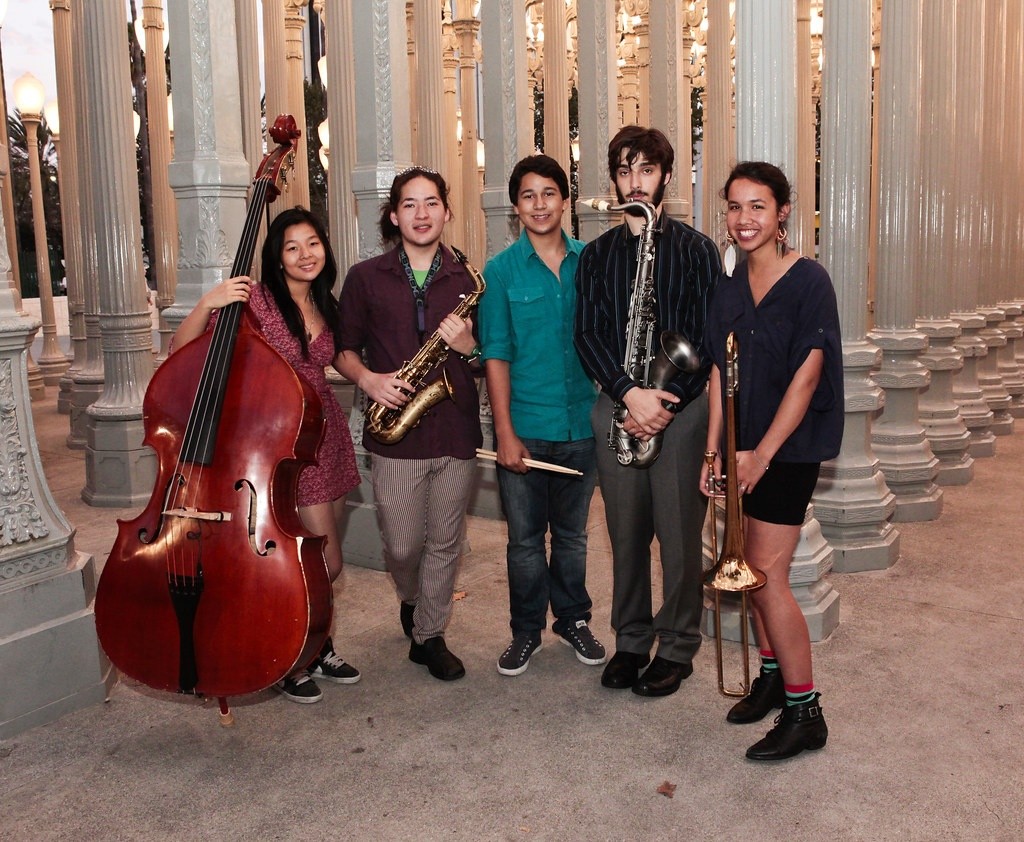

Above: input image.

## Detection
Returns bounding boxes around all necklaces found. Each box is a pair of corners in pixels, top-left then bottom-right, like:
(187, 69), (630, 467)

(305, 292), (315, 341)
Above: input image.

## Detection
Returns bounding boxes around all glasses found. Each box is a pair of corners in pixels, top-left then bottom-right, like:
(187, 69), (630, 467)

(395, 165), (439, 178)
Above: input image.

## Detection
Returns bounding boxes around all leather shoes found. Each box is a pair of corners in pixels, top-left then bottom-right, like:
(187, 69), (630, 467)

(726, 665), (784, 724)
(400, 600), (416, 638)
(631, 654), (694, 697)
(409, 634), (465, 680)
(600, 650), (651, 689)
(745, 691), (828, 760)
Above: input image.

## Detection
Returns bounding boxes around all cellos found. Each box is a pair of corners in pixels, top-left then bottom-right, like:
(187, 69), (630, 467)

(90, 108), (338, 729)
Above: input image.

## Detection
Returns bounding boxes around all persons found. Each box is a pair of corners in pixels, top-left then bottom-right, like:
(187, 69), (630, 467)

(571, 126), (722, 698)
(472, 154), (606, 676)
(330, 167), (483, 681)
(169, 209), (361, 703)
(701, 161), (846, 759)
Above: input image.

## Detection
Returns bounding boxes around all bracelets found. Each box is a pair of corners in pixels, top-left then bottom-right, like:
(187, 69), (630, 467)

(459, 343), (481, 363)
(753, 450), (769, 469)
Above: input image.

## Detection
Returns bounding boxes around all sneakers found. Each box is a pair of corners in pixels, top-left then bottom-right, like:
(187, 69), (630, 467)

(307, 639), (361, 684)
(497, 635), (543, 676)
(271, 668), (324, 703)
(558, 618), (607, 665)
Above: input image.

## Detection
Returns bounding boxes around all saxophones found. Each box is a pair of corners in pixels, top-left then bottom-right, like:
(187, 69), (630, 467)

(363, 243), (487, 446)
(578, 194), (702, 469)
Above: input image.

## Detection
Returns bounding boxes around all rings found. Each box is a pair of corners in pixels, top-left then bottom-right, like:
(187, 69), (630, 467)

(741, 487), (744, 492)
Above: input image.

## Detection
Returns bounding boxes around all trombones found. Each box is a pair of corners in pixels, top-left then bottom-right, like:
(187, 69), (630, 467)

(702, 331), (768, 697)
